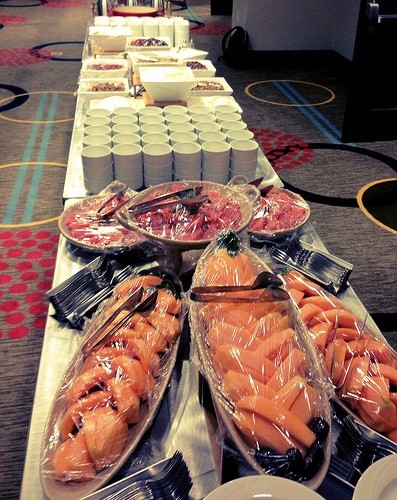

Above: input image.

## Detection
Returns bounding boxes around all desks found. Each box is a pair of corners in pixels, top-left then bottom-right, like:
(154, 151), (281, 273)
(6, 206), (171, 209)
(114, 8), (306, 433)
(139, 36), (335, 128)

(111, 6), (162, 16)
(20, 23), (397, 500)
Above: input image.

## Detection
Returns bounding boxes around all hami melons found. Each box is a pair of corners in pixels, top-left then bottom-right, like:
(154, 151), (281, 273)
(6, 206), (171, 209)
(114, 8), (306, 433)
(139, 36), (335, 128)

(283, 270), (397, 445)
(50, 275), (183, 480)
(199, 248), (317, 455)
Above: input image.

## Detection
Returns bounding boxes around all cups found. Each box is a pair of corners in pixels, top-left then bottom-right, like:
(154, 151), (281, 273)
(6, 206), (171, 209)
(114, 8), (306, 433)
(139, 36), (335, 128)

(94, 15), (190, 48)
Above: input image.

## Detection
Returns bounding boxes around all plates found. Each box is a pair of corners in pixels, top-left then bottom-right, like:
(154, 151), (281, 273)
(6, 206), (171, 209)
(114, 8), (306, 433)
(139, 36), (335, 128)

(38, 179), (397, 500)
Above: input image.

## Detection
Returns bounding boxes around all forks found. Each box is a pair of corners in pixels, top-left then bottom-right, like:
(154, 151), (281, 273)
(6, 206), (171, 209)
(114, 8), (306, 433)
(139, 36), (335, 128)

(101, 449), (192, 500)
(270, 246), (318, 278)
(74, 264), (132, 319)
(327, 454), (361, 486)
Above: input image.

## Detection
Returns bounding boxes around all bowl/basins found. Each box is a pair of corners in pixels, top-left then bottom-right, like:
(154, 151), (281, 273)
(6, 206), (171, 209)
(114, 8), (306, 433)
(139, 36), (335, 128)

(83, 105), (258, 182)
(88, 26), (132, 52)
(78, 36), (244, 114)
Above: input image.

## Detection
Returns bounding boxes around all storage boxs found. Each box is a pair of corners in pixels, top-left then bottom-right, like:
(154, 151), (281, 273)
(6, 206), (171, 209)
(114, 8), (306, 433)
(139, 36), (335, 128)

(183, 60), (216, 77)
(173, 47), (209, 60)
(190, 77), (233, 96)
(80, 58), (129, 78)
(129, 52), (180, 73)
(138, 67), (196, 101)
(78, 78), (129, 99)
(89, 26), (131, 52)
(126, 37), (172, 51)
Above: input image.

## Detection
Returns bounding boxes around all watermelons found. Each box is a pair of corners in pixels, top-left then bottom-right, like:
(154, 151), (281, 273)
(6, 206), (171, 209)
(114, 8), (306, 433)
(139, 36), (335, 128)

(245, 186), (306, 232)
(133, 182), (245, 241)
(63, 196), (148, 245)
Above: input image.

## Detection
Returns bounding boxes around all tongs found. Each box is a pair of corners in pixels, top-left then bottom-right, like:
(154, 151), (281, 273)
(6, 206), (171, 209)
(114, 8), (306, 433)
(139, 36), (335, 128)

(250, 175), (274, 194)
(81, 283), (158, 358)
(191, 270), (290, 303)
(96, 185), (137, 218)
(127, 185), (208, 217)
